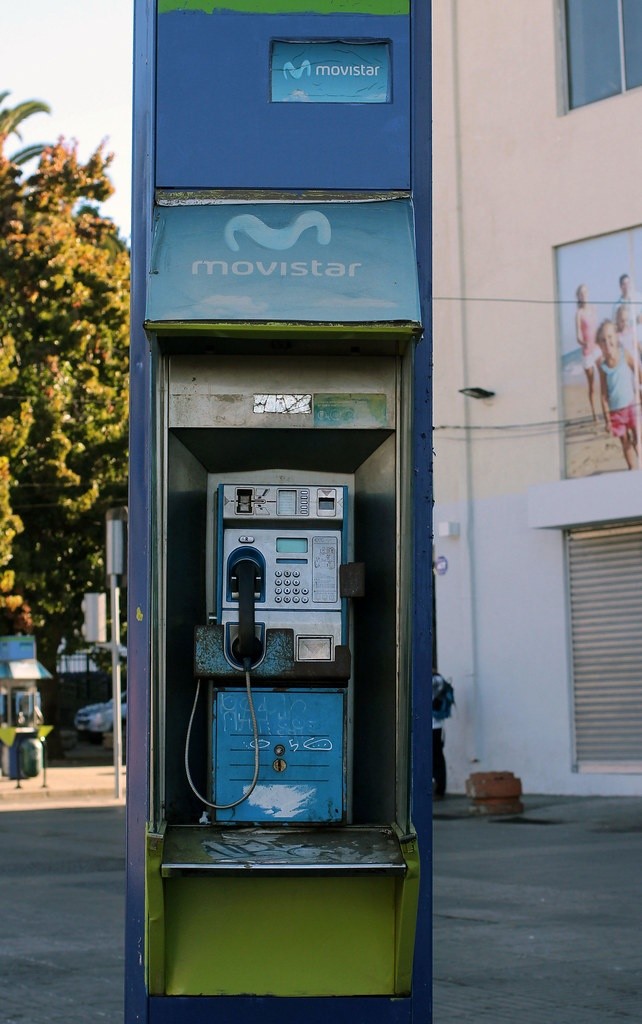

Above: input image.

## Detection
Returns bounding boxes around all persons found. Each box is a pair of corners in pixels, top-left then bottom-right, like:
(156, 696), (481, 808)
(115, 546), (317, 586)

(432, 663), (446, 802)
(575, 274), (642, 470)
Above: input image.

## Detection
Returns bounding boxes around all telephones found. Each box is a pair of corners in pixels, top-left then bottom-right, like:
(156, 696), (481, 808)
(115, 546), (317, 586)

(11, 692), (33, 721)
(206, 479), (353, 674)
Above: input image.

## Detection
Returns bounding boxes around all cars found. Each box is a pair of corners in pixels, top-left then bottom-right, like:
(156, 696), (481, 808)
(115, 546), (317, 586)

(73, 688), (128, 742)
